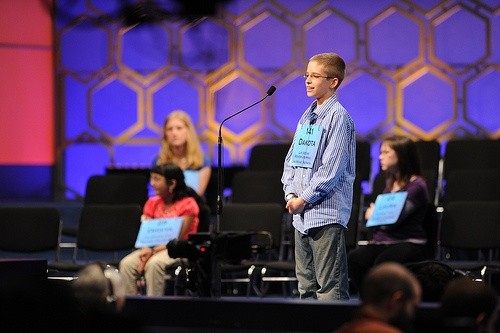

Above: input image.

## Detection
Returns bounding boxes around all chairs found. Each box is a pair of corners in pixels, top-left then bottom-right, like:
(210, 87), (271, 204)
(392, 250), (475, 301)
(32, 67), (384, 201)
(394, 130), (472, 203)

(0, 136), (500, 298)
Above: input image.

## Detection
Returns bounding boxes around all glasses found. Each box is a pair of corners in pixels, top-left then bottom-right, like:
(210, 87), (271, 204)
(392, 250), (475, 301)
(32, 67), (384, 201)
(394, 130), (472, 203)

(304, 72), (336, 78)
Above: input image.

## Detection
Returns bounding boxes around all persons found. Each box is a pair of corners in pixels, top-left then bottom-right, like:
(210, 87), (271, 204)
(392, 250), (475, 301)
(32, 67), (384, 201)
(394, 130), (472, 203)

(281, 52), (356, 301)
(147, 110), (211, 201)
(348, 136), (433, 276)
(334, 262), (422, 333)
(120, 162), (199, 297)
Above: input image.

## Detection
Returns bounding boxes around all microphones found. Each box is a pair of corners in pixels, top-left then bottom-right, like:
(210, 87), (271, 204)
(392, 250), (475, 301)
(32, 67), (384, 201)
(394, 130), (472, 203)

(216, 85), (276, 215)
